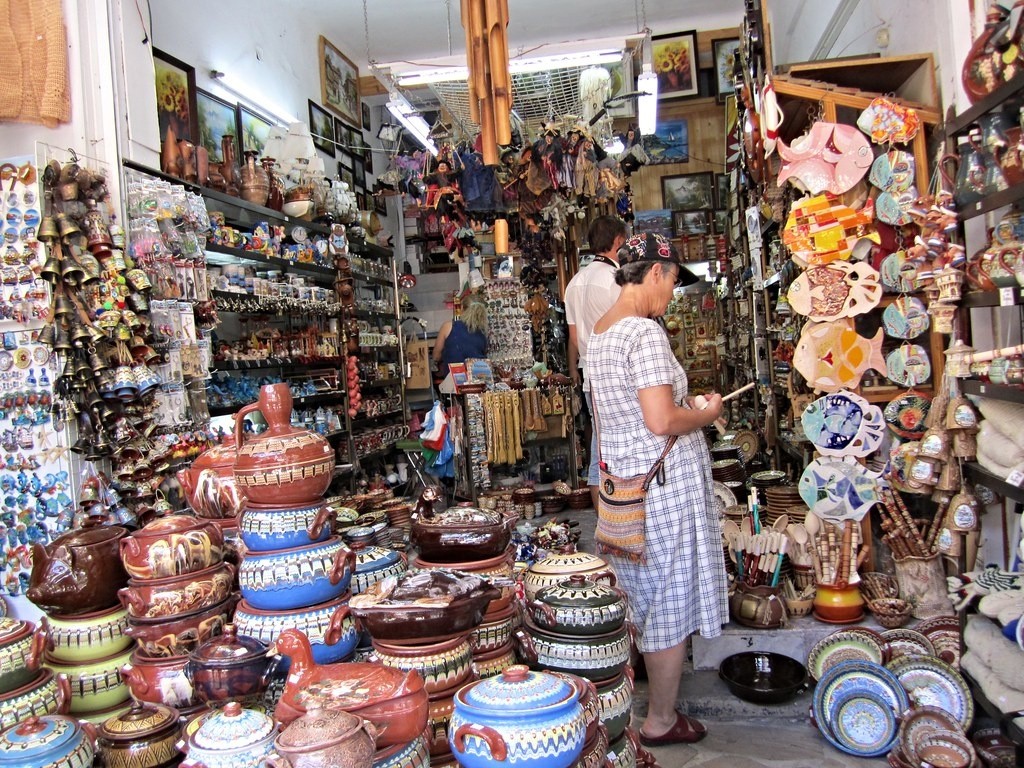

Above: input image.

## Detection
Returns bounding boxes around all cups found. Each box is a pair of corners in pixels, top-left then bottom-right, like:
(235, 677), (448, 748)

(208, 212), (313, 264)
(339, 424), (410, 458)
(361, 394), (402, 417)
(209, 265), (335, 304)
(357, 320), (398, 345)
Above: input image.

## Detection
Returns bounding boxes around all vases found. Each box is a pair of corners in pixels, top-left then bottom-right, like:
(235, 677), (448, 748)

(220, 134), (286, 211)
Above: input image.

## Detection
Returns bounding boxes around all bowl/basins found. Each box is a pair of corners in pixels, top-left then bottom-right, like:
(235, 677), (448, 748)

(282, 201), (309, 217)
(783, 561), (816, 618)
(476, 481), (590, 519)
(327, 489), (412, 553)
(860, 571), (913, 628)
(720, 651), (807, 705)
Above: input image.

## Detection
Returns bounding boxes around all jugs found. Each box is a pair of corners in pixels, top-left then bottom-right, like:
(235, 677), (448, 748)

(232, 382), (336, 505)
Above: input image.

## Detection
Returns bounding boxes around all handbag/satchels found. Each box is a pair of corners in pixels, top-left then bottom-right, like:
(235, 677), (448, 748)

(591, 466), (648, 568)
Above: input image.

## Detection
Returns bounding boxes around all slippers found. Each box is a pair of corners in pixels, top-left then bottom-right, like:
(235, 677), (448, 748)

(639, 708), (710, 747)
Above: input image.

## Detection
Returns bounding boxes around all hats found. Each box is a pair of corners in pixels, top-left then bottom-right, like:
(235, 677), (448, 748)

(615, 231), (700, 288)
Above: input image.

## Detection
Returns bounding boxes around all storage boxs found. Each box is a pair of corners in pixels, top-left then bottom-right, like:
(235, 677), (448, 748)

(403, 217), (428, 275)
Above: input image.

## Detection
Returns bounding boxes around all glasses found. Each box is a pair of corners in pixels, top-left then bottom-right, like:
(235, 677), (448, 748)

(650, 262), (683, 289)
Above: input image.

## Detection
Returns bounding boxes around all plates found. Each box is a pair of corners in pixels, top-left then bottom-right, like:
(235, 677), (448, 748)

(709, 429), (810, 583)
(809, 615), (1017, 768)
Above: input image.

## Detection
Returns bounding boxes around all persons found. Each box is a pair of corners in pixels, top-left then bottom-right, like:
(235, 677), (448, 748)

(901, 190), (966, 286)
(433, 303), (487, 375)
(587, 232), (724, 745)
(565, 217), (630, 513)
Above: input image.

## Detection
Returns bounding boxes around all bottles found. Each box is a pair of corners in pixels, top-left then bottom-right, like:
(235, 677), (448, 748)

(961, 0), (1024, 104)
(359, 361), (399, 381)
(353, 295), (394, 313)
(348, 252), (393, 281)
(163, 128), (335, 227)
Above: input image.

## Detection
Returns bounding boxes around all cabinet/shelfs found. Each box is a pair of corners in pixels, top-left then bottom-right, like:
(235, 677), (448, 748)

(943, 69), (1024, 508)
(180, 185), (407, 475)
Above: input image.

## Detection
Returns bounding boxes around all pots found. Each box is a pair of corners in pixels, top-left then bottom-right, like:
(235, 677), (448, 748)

(0, 513), (661, 768)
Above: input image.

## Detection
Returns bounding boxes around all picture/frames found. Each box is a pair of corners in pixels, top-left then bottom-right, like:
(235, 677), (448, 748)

(651, 29), (700, 102)
(659, 169), (729, 236)
(710, 36), (741, 105)
(307, 35), (387, 217)
(151, 47), (200, 145)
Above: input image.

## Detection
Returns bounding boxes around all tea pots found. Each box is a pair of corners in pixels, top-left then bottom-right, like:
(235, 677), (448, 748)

(939, 105), (1024, 289)
(969, 353), (1024, 387)
